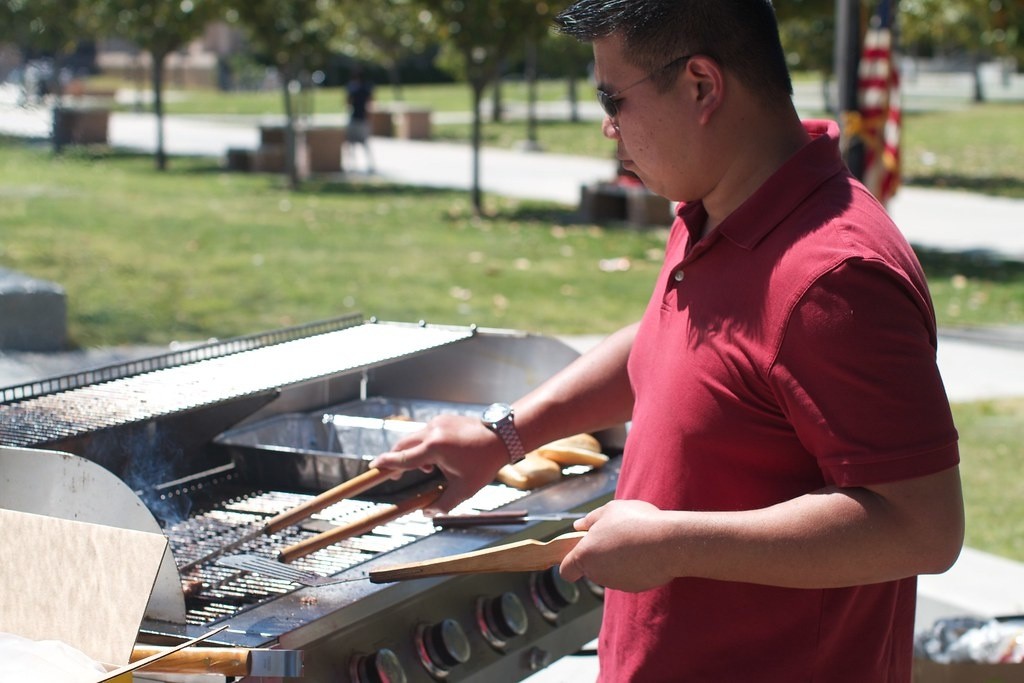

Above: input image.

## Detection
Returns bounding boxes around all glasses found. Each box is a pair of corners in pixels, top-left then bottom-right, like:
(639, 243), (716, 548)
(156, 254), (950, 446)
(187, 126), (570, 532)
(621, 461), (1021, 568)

(595, 54), (693, 117)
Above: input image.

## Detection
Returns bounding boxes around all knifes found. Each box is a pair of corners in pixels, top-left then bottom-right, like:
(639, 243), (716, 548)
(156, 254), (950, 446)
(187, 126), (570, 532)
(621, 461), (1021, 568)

(433, 509), (592, 526)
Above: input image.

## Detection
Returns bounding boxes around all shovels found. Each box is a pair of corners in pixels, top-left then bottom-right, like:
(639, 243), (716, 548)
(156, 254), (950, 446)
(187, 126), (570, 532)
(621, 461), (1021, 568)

(213, 528), (589, 587)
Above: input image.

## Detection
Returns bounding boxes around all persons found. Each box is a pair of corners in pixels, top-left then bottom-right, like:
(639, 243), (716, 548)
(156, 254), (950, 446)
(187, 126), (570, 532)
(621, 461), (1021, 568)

(368, 0), (965, 683)
(338, 65), (377, 173)
(18, 57), (90, 108)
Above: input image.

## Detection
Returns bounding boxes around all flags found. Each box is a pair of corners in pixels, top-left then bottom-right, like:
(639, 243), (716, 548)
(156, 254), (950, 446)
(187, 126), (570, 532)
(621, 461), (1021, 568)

(844, 0), (901, 213)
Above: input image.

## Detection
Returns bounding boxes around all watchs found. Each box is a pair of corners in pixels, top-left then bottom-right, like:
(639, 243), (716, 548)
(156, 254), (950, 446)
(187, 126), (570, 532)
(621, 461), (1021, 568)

(480, 402), (526, 465)
